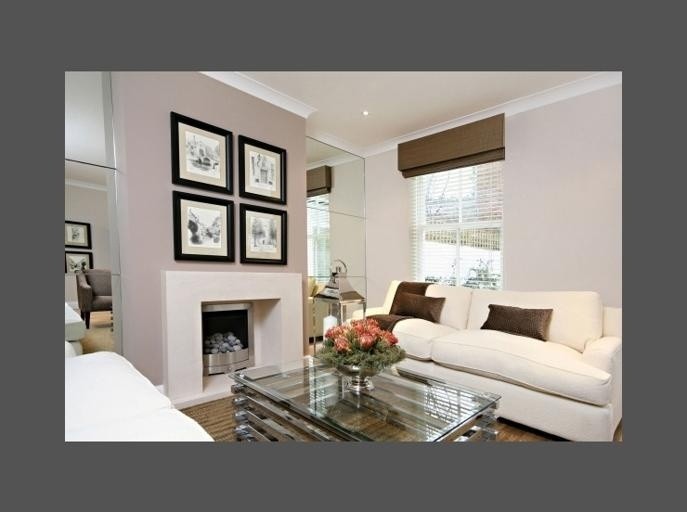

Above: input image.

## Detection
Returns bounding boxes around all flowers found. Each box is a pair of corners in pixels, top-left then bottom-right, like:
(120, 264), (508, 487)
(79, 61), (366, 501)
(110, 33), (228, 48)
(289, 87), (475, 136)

(313, 319), (406, 368)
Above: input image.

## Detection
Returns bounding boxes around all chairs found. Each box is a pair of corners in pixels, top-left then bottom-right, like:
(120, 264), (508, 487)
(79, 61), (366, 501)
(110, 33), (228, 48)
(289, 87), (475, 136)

(77, 269), (111, 329)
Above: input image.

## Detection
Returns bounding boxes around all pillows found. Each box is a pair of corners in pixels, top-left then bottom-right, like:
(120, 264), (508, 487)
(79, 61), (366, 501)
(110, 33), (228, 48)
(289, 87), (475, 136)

(467, 292), (603, 350)
(480, 304), (553, 344)
(393, 293), (445, 323)
(383, 280), (474, 331)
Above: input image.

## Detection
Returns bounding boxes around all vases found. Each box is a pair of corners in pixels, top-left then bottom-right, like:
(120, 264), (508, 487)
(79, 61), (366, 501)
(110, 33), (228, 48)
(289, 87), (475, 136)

(335, 362), (376, 395)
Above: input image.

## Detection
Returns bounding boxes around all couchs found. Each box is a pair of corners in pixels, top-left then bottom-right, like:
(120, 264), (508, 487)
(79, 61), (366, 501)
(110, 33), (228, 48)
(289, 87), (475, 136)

(65, 300), (86, 357)
(65, 351), (217, 441)
(353, 284), (623, 444)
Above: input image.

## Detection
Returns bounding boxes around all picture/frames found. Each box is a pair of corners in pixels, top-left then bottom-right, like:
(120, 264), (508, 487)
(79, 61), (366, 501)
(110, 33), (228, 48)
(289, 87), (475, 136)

(65, 221), (91, 248)
(65, 251), (93, 274)
(172, 190), (234, 263)
(170, 111), (233, 194)
(238, 203), (287, 265)
(237, 133), (287, 205)
